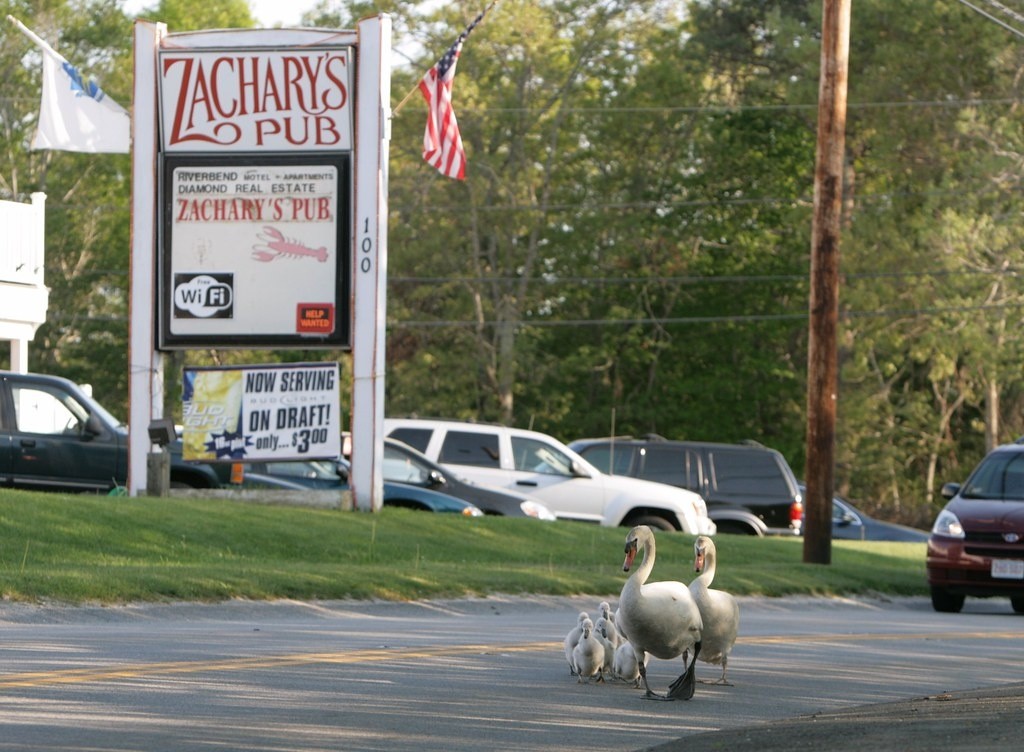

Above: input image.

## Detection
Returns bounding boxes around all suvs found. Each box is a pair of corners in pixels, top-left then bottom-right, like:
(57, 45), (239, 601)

(1, 369), (222, 500)
(380, 419), (721, 539)
(530, 430), (804, 541)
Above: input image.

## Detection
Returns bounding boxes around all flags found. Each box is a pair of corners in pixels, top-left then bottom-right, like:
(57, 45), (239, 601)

(13, 19), (130, 155)
(418, 8), (483, 181)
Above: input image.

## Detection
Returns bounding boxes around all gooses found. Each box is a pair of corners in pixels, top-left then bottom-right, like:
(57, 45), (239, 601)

(615, 524), (704, 700)
(564, 611), (589, 674)
(594, 602), (649, 690)
(573, 621), (605, 685)
(688, 536), (738, 686)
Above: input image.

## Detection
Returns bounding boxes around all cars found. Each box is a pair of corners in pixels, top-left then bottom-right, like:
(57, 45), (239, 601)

(152, 419), (557, 525)
(796, 485), (934, 546)
(924, 432), (1024, 613)
